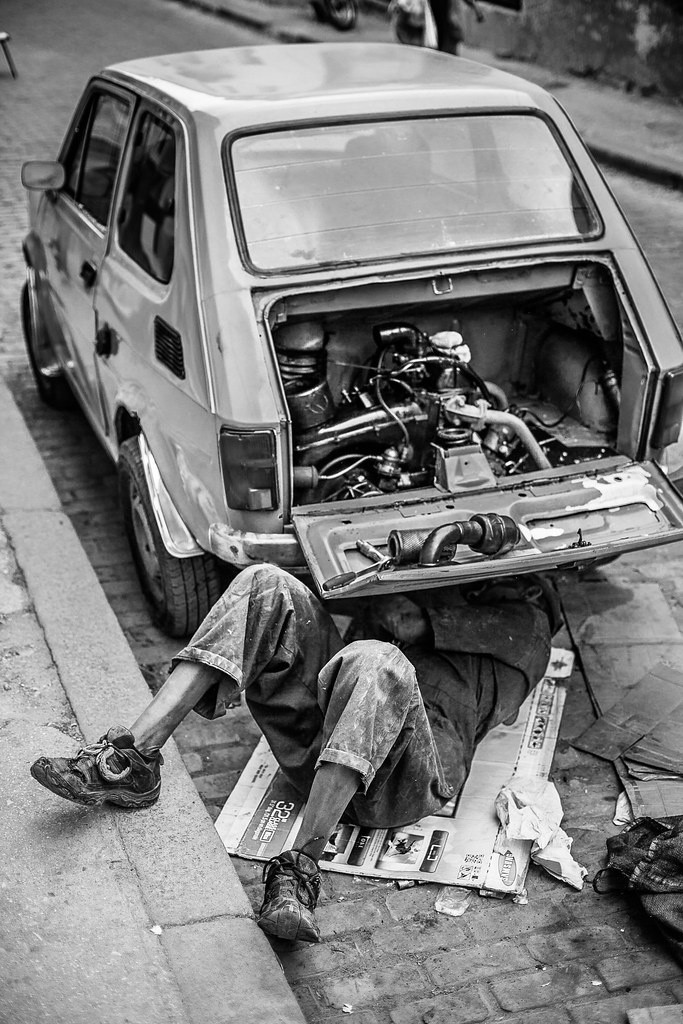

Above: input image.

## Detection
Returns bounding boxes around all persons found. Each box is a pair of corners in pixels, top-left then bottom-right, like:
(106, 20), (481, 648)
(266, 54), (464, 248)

(29, 561), (567, 940)
(396, 0), (484, 57)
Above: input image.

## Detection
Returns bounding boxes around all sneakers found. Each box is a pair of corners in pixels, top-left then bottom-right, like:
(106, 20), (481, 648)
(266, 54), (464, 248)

(258, 836), (327, 943)
(30, 725), (164, 810)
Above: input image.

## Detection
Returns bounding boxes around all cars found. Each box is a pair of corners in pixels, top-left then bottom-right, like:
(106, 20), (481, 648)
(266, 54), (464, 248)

(19, 41), (682, 639)
